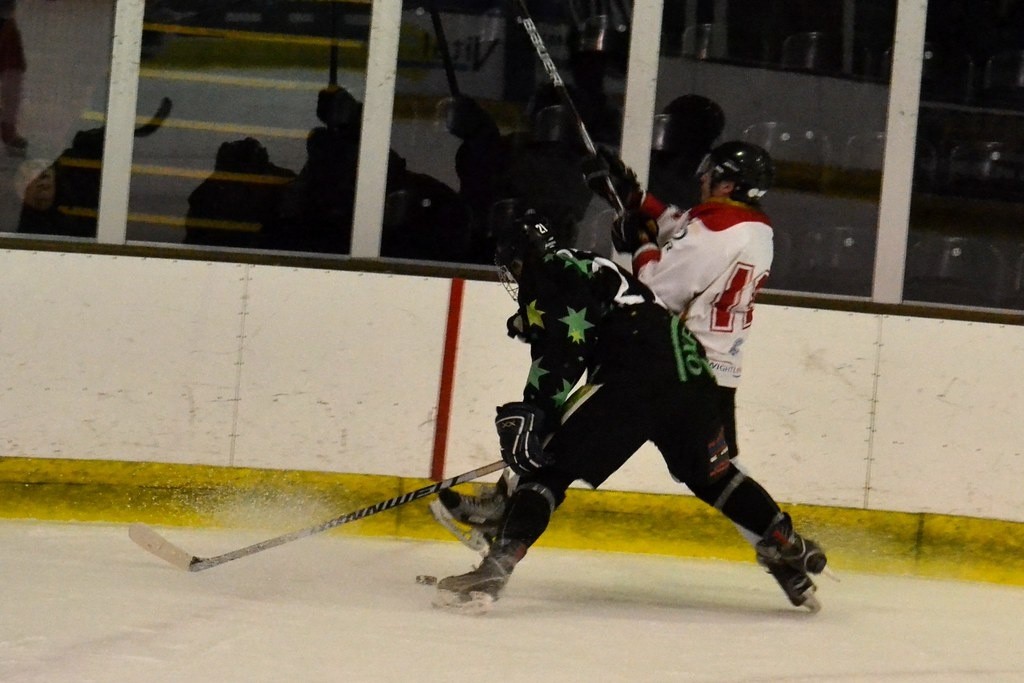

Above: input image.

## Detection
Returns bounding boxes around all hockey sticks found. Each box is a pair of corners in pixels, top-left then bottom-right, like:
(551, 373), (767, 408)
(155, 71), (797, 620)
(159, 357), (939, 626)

(514, 1), (628, 219)
(326, 2), (346, 87)
(427, 2), (465, 100)
(128, 459), (508, 573)
(134, 96), (175, 139)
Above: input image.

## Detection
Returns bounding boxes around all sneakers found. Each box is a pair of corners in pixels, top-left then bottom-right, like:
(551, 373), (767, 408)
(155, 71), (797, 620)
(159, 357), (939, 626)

(431, 469), (519, 553)
(414, 538), (526, 617)
(755, 512), (828, 614)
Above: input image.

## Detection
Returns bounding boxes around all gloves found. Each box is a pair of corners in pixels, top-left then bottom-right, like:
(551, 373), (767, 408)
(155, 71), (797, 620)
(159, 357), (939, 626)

(581, 144), (642, 211)
(610, 212), (657, 253)
(494, 402), (567, 488)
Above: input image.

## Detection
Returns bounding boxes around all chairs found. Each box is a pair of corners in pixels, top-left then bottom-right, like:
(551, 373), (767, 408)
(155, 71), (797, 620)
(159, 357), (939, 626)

(476, 0), (1023, 312)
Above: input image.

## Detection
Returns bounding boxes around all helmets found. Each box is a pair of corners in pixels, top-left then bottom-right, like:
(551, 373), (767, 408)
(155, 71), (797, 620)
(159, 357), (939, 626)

(493, 211), (570, 303)
(651, 96), (725, 154)
(698, 140), (773, 203)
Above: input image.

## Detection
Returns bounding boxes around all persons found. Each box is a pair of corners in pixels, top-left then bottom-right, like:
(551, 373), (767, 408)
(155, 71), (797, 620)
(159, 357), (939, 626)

(0, 0), (722, 265)
(430, 143), (845, 614)
